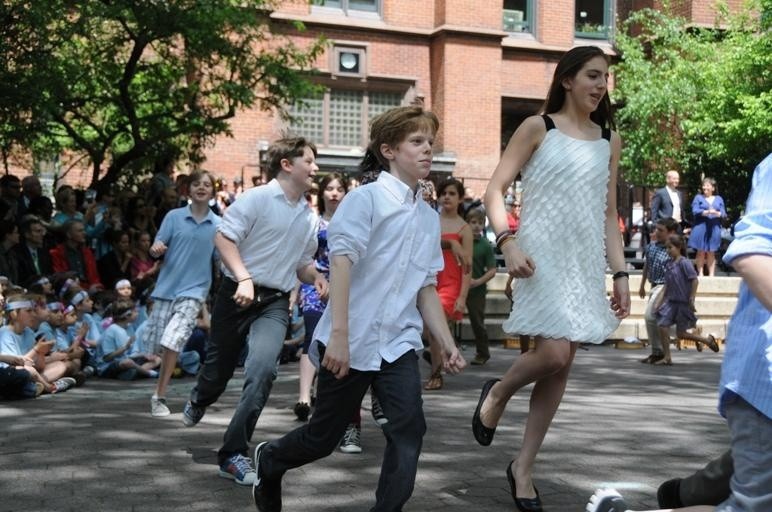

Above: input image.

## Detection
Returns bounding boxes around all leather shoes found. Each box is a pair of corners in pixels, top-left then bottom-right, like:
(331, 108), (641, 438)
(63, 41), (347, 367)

(507, 461), (543, 512)
(472, 379), (502, 446)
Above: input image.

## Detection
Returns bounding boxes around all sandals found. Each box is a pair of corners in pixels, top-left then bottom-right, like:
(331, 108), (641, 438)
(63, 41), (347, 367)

(708, 335), (721, 352)
(425, 372), (443, 390)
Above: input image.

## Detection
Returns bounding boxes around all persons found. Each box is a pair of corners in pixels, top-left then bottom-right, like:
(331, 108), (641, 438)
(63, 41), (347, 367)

(1, 171), (251, 404)
(657, 446), (733, 509)
(180, 136), (330, 485)
(472, 45), (633, 510)
(585, 153), (770, 510)
(147, 169), (222, 414)
(615, 166), (729, 366)
(251, 108), (467, 511)
(278, 169), (523, 453)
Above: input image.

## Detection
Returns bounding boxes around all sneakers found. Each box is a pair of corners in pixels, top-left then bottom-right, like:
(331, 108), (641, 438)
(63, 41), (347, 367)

(252, 442), (282, 512)
(339, 422), (362, 452)
(152, 397), (171, 416)
(220, 453), (256, 485)
(694, 326), (703, 351)
(471, 353), (485, 366)
(51, 377), (77, 393)
(183, 400), (205, 427)
(295, 402), (309, 422)
(370, 386), (388, 428)
(658, 477), (681, 509)
(642, 352), (672, 364)
(586, 488), (627, 511)
